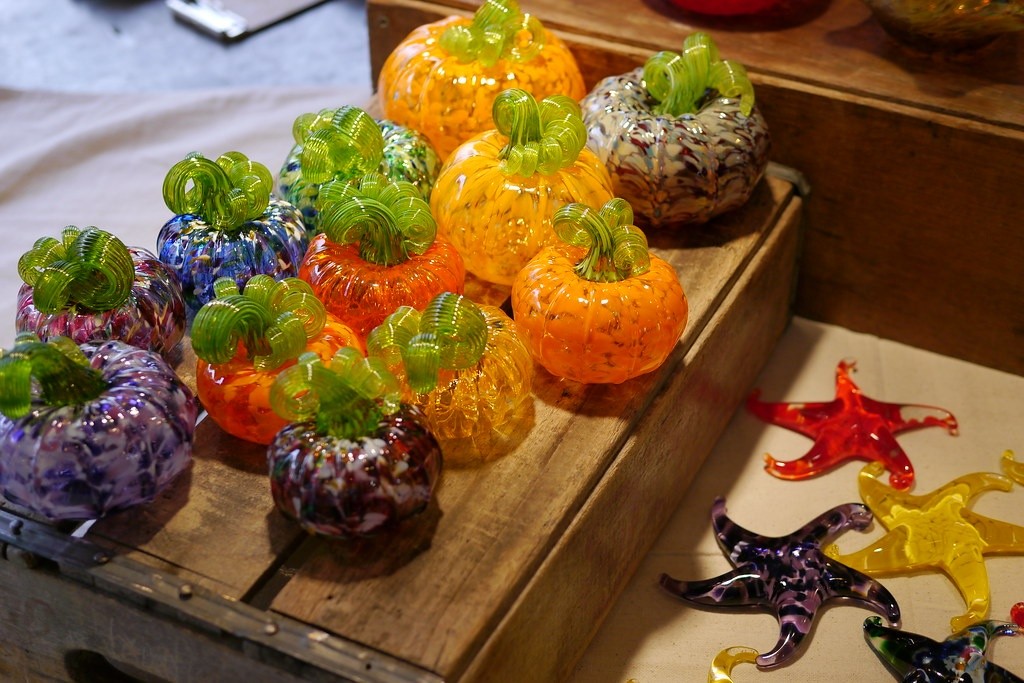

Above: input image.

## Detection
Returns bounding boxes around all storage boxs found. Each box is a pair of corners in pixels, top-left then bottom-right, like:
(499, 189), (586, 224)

(0, 162), (812, 683)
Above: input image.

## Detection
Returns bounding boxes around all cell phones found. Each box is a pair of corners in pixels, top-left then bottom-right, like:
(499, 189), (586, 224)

(165, 0), (325, 43)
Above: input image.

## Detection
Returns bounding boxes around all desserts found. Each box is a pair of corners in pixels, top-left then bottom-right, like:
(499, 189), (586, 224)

(663, 352), (1024, 683)
(0, 0), (768, 544)
